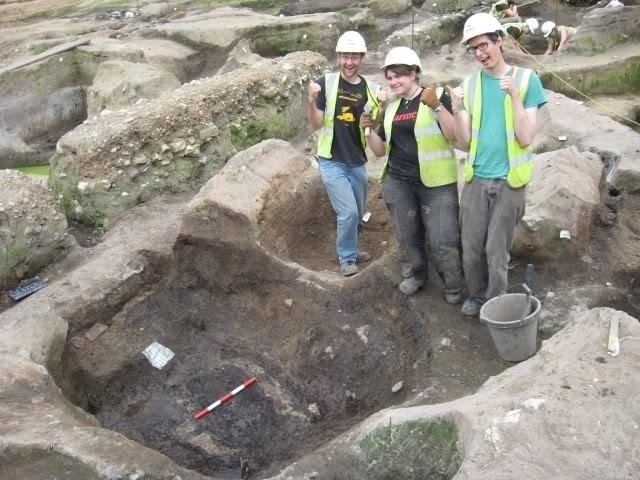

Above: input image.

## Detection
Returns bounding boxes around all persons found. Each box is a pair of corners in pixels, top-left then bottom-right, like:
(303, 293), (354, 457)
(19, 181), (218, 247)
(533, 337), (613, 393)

(443, 12), (546, 317)
(501, 17), (539, 41)
(490, 0), (523, 25)
(306, 30), (380, 278)
(541, 21), (576, 56)
(355, 45), (465, 305)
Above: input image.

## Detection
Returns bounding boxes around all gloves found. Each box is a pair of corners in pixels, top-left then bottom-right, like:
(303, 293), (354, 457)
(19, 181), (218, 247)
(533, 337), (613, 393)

(420, 84), (444, 112)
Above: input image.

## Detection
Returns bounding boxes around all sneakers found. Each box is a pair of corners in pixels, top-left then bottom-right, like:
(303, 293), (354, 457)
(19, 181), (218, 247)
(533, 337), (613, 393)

(460, 295), (490, 316)
(340, 261), (358, 276)
(444, 288), (463, 304)
(336, 252), (371, 263)
(398, 273), (425, 296)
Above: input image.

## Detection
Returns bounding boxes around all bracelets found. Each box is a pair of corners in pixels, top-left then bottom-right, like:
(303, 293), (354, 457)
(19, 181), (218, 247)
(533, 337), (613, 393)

(433, 102), (444, 114)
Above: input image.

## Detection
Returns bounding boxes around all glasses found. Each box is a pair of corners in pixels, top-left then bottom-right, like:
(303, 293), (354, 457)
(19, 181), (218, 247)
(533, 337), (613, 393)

(466, 40), (492, 54)
(340, 55), (361, 61)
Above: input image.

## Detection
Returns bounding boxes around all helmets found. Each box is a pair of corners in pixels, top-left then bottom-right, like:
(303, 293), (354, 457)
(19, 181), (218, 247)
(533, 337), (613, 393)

(335, 31), (367, 53)
(541, 21), (555, 38)
(380, 47), (422, 73)
(526, 18), (539, 34)
(460, 12), (506, 46)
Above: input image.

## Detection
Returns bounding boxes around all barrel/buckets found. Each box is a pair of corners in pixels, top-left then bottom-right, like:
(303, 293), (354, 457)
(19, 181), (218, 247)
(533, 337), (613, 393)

(476, 292), (544, 364)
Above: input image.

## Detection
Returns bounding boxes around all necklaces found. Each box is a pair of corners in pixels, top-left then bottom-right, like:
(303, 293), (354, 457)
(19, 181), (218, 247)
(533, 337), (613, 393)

(400, 84), (422, 110)
